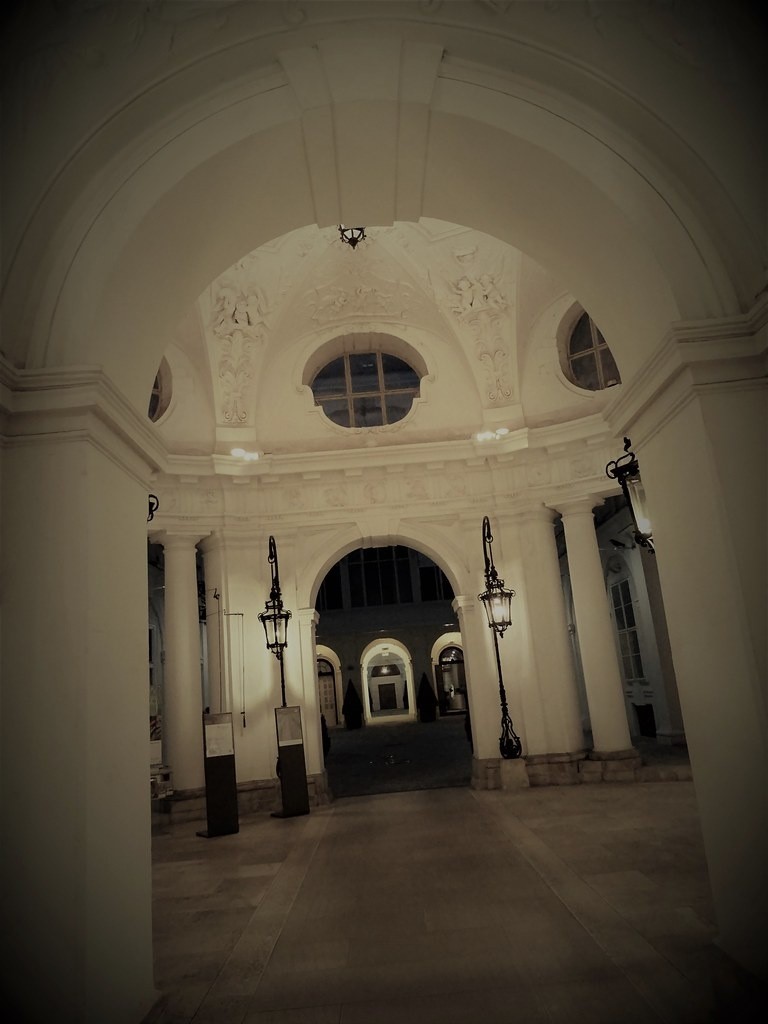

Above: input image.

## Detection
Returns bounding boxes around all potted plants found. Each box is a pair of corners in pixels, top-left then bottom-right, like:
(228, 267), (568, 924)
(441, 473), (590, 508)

(342, 679), (363, 730)
(415, 672), (439, 723)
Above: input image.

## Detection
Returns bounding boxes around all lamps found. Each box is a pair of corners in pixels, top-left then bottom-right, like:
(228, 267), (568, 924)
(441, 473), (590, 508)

(258, 535), (293, 708)
(605, 436), (657, 554)
(338, 224), (367, 250)
(476, 516), (522, 756)
